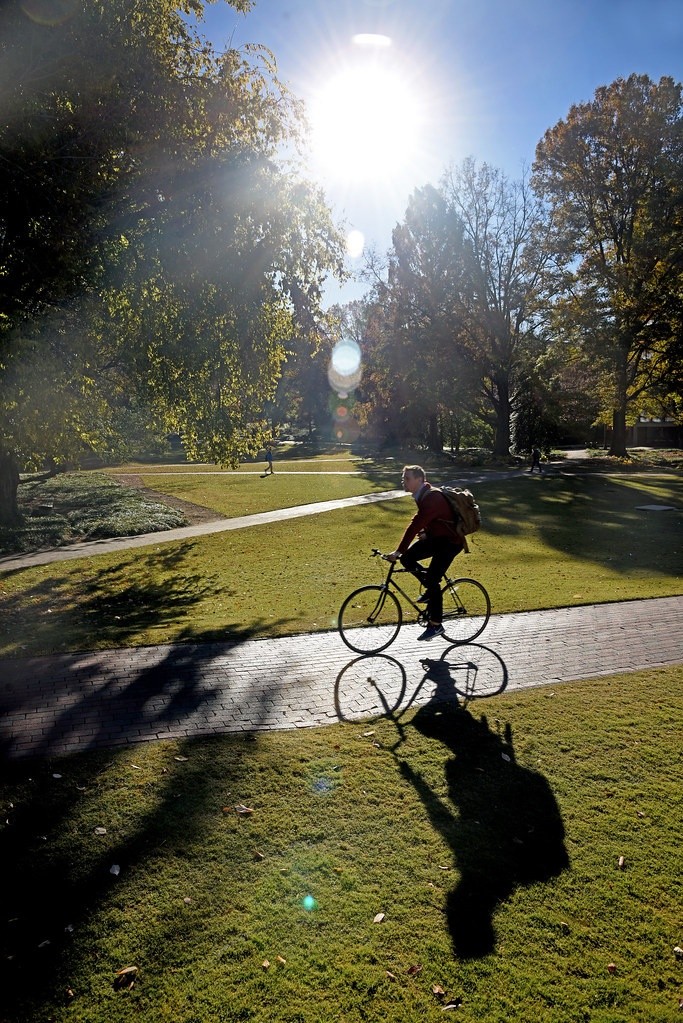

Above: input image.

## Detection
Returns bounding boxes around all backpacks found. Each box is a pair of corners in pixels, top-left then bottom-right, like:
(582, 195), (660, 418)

(420, 485), (482, 536)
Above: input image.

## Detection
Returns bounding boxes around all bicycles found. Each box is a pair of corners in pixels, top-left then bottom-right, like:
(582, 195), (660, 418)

(337, 548), (491, 655)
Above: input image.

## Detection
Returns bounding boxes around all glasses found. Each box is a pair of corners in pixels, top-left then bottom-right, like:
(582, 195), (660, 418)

(400, 476), (416, 482)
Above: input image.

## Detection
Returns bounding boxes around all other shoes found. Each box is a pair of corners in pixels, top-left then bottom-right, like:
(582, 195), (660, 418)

(417, 583), (442, 603)
(417, 620), (446, 641)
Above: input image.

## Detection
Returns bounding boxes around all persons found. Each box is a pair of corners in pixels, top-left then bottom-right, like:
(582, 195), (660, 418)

(528, 445), (543, 473)
(385, 465), (465, 642)
(264, 447), (274, 474)
